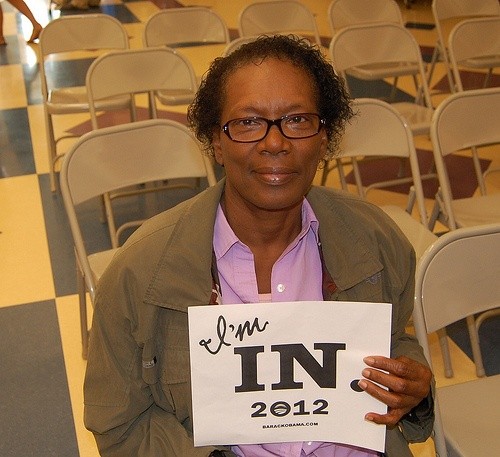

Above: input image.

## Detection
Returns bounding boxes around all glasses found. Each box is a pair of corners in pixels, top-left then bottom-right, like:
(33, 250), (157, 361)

(216, 113), (327, 143)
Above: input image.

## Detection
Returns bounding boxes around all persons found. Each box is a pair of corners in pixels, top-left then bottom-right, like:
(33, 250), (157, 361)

(82, 30), (436, 457)
(0, 0), (43, 46)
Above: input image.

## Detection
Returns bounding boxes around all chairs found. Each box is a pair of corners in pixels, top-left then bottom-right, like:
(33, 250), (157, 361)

(39, 0), (500, 456)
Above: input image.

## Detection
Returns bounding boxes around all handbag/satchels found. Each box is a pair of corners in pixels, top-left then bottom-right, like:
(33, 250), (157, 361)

(51, 0), (100, 10)
